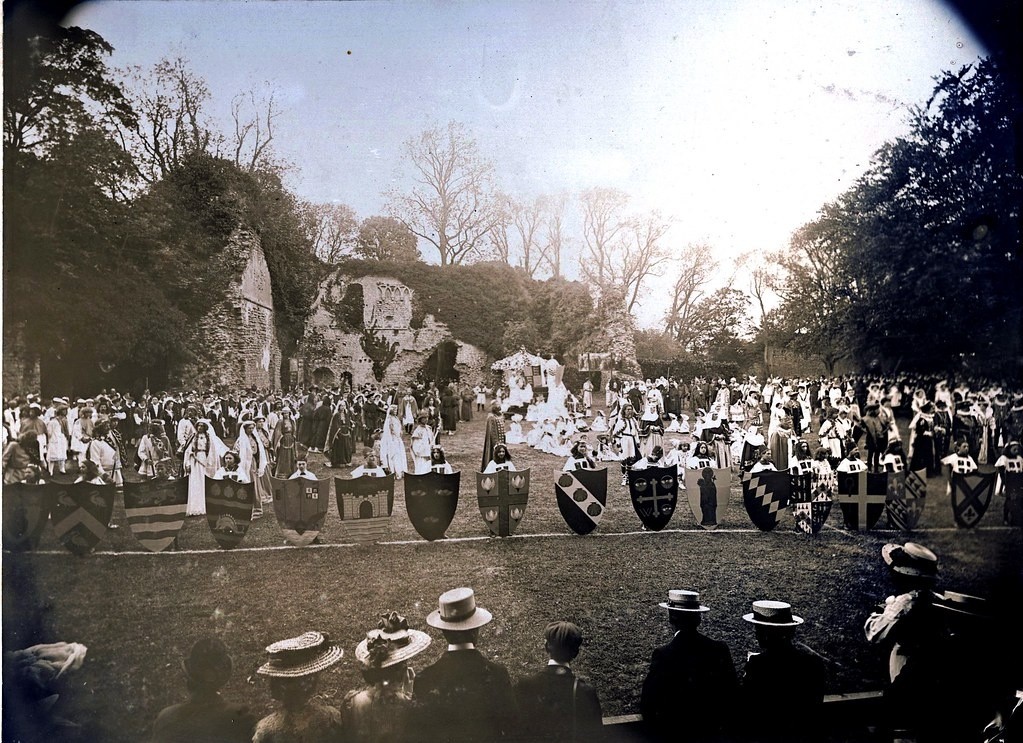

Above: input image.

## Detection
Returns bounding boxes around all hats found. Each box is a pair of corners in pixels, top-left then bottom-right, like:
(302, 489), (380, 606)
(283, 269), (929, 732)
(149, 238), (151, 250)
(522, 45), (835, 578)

(931, 590), (987, 616)
(659, 590), (711, 612)
(257, 631), (343, 678)
(545, 622), (584, 661)
(426, 586), (491, 631)
(882, 542), (943, 579)
(742, 600), (804, 626)
(182, 637), (233, 690)
(355, 611), (433, 670)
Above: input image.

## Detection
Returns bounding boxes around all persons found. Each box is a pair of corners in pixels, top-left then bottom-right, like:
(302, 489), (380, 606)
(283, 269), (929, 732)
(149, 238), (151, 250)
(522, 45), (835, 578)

(2, 641), (98, 743)
(637, 588), (742, 743)
(411, 588), (523, 743)
(865, 542), (991, 743)
(343, 612), (433, 743)
(739, 600), (827, 743)
(247, 632), (342, 743)
(3, 366), (1023, 552)
(514, 620), (604, 743)
(149, 636), (259, 743)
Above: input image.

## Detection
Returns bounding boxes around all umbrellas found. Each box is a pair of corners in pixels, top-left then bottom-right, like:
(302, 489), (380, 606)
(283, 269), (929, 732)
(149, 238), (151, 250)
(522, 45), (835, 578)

(491, 347), (547, 372)
